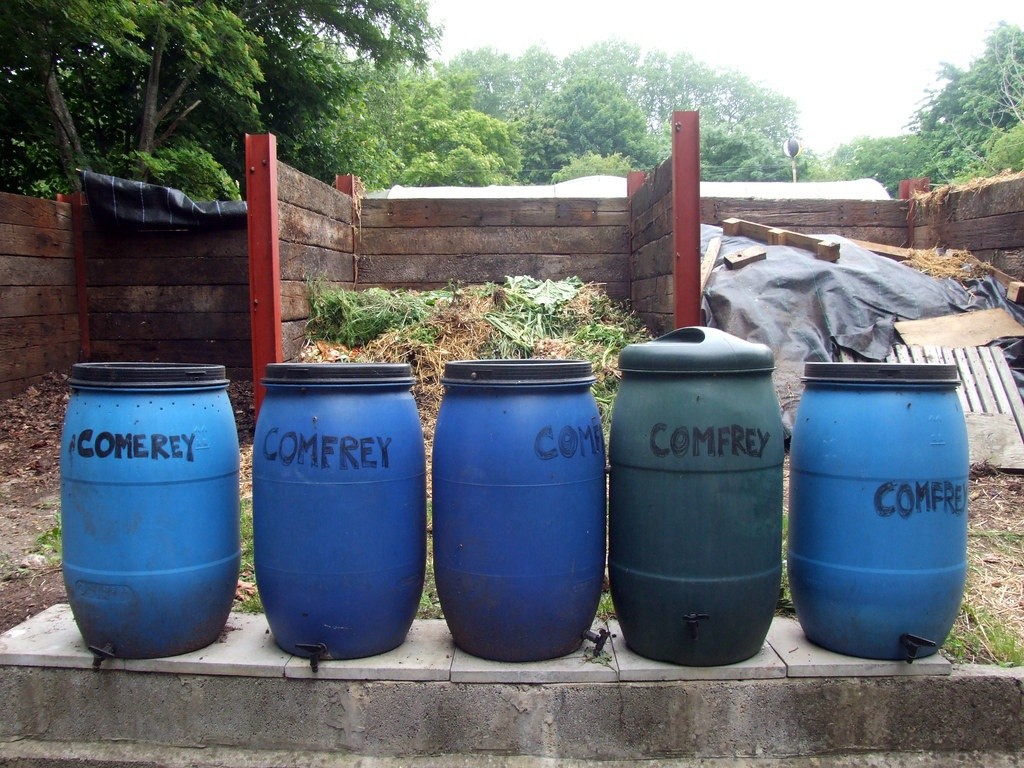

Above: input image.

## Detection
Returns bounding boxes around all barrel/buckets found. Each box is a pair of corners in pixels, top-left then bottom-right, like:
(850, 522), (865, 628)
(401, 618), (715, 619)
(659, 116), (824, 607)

(251, 363), (427, 674)
(59, 361), (239, 674)
(609, 326), (784, 666)
(786, 361), (969, 664)
(432, 359), (610, 662)
